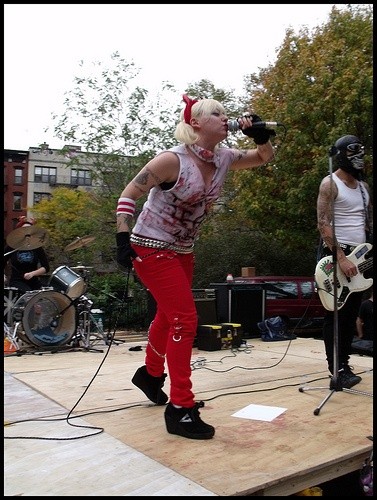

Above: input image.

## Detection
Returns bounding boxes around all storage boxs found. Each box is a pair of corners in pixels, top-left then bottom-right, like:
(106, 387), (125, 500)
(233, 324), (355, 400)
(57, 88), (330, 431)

(198, 324), (221, 351)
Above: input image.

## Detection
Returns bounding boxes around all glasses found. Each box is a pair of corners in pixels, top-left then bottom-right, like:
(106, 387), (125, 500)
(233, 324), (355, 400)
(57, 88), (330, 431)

(345, 143), (366, 153)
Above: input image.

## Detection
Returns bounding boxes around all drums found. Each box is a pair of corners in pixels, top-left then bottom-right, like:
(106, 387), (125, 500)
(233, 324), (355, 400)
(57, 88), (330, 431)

(48, 265), (84, 301)
(11, 287), (78, 349)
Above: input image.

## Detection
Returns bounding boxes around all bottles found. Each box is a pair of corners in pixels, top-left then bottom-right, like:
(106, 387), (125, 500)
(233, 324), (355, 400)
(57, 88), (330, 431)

(226, 274), (233, 283)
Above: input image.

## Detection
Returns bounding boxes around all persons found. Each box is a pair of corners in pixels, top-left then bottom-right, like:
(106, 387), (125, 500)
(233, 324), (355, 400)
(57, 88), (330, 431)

(115, 95), (274, 440)
(316, 134), (370, 390)
(3, 215), (50, 295)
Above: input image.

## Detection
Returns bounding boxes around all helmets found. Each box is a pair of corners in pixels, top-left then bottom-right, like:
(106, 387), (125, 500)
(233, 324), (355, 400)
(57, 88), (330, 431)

(335, 136), (368, 181)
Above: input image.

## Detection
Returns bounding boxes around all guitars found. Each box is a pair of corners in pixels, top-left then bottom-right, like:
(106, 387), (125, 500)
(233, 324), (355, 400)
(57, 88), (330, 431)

(314, 243), (374, 311)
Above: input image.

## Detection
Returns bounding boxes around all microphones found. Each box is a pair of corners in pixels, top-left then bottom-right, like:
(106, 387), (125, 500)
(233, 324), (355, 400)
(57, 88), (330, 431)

(228, 121), (284, 131)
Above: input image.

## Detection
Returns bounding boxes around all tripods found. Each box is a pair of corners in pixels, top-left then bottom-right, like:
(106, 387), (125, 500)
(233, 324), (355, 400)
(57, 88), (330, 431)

(77, 298), (125, 353)
(298, 157), (373, 416)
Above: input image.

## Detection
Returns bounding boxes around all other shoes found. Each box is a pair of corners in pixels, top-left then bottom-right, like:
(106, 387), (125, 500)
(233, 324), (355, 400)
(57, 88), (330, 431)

(164, 401), (215, 440)
(331, 364), (362, 389)
(132, 366), (168, 405)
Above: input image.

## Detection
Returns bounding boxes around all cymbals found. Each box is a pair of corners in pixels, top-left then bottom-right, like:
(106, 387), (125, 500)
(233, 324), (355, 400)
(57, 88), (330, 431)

(7, 226), (50, 250)
(64, 234), (97, 252)
(70, 265), (94, 269)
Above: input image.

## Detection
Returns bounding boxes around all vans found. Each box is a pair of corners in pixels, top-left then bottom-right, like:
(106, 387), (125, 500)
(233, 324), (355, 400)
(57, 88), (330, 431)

(233, 276), (328, 321)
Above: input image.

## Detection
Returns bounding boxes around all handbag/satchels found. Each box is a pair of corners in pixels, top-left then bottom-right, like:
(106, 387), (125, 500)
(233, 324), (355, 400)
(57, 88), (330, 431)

(259, 315), (298, 342)
